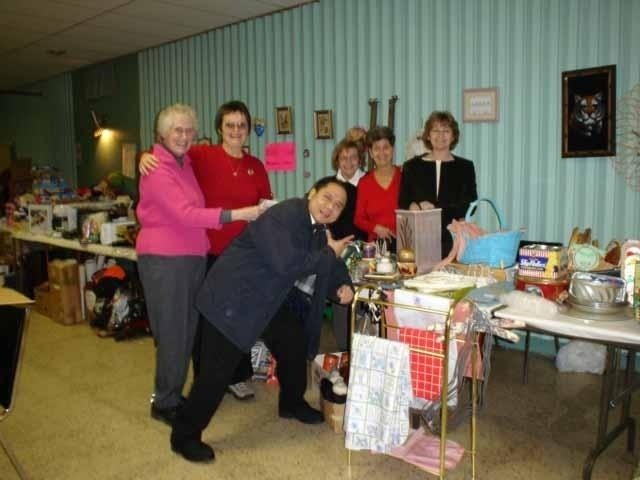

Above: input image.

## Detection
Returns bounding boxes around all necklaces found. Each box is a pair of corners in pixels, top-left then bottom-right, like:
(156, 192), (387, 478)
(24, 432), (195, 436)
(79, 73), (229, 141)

(224, 149), (242, 177)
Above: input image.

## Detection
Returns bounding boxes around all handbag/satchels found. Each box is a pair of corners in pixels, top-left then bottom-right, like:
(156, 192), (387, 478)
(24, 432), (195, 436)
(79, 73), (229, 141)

(447, 198), (519, 268)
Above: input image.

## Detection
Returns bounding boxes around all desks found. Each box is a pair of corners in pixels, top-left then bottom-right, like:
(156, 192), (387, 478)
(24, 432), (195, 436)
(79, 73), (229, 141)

(0, 225), (138, 298)
(354, 270), (640, 480)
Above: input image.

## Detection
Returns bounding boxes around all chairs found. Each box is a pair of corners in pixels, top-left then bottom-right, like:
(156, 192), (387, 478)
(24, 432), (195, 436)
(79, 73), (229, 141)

(0, 305), (31, 480)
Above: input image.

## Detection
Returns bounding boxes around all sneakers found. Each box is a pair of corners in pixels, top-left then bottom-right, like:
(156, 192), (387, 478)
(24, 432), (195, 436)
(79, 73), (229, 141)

(150, 399), (187, 427)
(228, 382), (256, 401)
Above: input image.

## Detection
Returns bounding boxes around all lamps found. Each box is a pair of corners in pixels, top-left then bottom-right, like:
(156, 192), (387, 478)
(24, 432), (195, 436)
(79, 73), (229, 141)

(89, 110), (105, 138)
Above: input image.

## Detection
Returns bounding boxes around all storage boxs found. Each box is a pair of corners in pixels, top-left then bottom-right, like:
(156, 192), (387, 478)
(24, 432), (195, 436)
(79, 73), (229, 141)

(34, 259), (81, 326)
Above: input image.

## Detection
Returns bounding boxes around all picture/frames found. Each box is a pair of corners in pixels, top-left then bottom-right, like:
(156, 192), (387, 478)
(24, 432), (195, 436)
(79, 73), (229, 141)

(462, 88), (499, 124)
(313, 109), (333, 140)
(273, 105), (292, 135)
(561, 64), (617, 158)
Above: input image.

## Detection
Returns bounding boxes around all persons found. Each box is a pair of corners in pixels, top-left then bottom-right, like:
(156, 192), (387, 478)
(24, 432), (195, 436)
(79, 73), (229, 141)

(171, 177), (355, 463)
(353, 126), (402, 253)
(399, 111), (477, 261)
(331, 139), (367, 352)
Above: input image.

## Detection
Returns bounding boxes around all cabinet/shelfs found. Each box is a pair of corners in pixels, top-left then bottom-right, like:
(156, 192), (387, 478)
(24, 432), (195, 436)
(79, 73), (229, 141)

(344, 282), (478, 480)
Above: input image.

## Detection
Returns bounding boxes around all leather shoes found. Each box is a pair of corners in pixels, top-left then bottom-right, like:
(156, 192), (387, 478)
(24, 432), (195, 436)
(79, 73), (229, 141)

(278, 397), (325, 424)
(170, 441), (216, 465)
